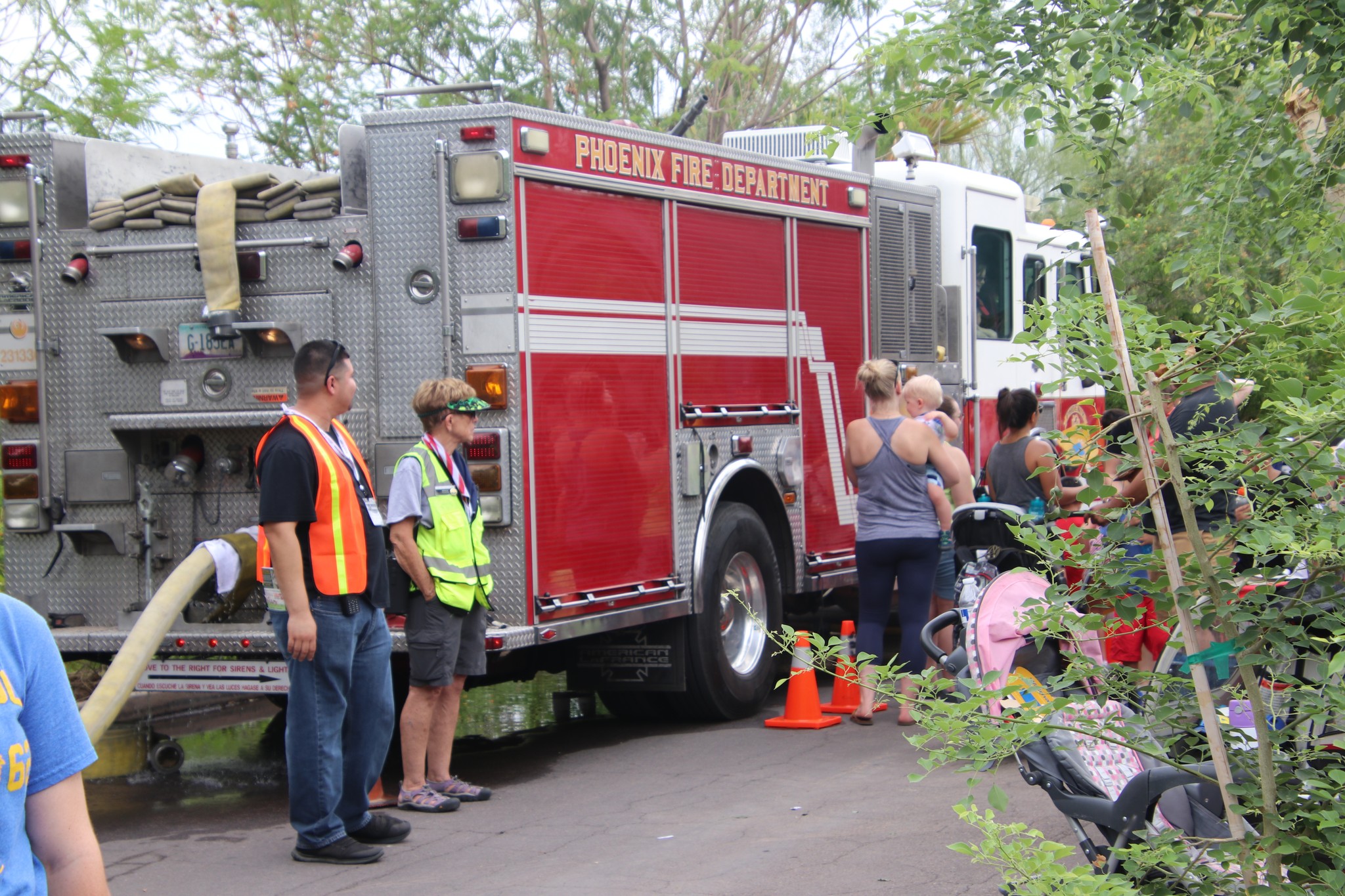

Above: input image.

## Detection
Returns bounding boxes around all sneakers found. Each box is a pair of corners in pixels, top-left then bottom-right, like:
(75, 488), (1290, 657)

(348, 813), (412, 843)
(291, 834), (384, 864)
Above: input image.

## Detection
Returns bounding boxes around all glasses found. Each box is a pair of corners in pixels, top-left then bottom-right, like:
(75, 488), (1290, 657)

(890, 359), (899, 387)
(324, 340), (346, 386)
(950, 414), (965, 422)
(1035, 404), (1043, 414)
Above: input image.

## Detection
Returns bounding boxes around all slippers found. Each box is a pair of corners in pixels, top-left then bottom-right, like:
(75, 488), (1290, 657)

(850, 709), (873, 725)
(898, 720), (917, 726)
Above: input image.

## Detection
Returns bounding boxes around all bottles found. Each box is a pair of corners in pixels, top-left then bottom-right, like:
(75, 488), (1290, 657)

(978, 493), (992, 503)
(959, 577), (980, 626)
(1030, 497), (1045, 521)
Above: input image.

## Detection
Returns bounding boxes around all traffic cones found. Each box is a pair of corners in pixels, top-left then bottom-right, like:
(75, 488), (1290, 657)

(763, 630), (842, 730)
(819, 620), (888, 714)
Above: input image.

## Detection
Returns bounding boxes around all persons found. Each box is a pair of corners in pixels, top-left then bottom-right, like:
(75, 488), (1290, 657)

(256, 339), (412, 863)
(976, 256), (998, 339)
(0, 592), (113, 896)
(845, 332), (1345, 802)
(384, 377), (493, 811)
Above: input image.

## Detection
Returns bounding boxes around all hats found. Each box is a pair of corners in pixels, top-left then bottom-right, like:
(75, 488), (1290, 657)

(417, 397), (490, 418)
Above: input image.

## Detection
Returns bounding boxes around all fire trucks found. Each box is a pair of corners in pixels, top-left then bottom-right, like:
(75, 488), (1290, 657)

(2, 77), (1110, 730)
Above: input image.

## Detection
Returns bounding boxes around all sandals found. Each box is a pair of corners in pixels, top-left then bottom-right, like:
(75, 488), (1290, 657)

(397, 779), (461, 811)
(426, 775), (490, 801)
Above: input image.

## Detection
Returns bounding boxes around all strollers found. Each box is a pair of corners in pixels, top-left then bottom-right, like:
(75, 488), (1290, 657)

(919, 505), (1289, 896)
